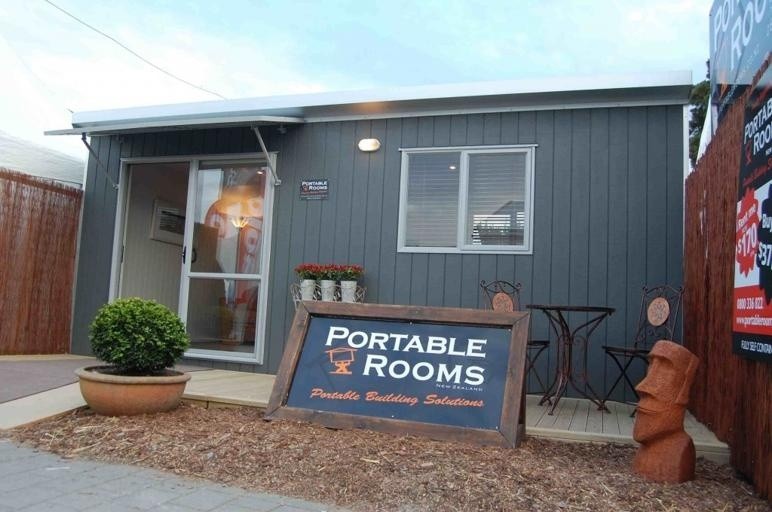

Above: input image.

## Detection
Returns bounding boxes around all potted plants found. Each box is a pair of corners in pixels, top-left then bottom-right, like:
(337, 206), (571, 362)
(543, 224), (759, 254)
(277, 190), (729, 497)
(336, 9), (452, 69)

(75, 297), (192, 418)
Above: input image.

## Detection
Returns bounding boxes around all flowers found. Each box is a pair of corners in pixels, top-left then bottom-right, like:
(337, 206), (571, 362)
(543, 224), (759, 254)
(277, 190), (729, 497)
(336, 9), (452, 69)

(295, 263), (364, 280)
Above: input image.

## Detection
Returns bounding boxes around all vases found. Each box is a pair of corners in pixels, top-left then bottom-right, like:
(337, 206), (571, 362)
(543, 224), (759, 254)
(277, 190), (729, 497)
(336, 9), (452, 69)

(299, 279), (358, 303)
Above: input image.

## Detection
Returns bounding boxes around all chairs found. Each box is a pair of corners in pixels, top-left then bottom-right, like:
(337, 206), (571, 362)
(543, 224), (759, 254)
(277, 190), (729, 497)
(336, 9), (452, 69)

(478, 279), (553, 408)
(597, 284), (686, 417)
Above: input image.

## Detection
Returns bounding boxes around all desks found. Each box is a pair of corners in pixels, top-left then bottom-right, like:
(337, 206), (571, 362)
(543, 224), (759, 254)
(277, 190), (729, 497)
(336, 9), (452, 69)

(525, 304), (617, 415)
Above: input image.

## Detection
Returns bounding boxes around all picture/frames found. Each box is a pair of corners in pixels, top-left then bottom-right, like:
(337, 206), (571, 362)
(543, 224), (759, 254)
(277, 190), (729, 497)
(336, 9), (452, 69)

(149, 197), (186, 245)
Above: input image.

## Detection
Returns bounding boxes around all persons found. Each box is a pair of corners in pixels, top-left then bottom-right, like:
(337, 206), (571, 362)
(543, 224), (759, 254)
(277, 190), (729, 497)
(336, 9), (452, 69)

(633, 339), (702, 485)
